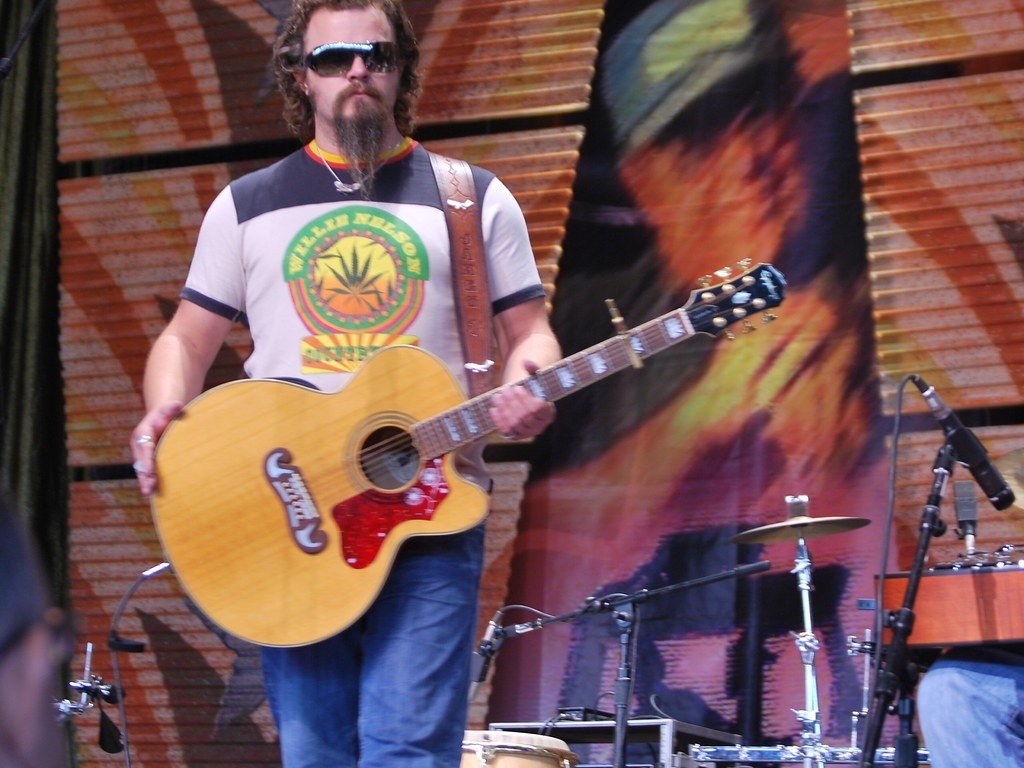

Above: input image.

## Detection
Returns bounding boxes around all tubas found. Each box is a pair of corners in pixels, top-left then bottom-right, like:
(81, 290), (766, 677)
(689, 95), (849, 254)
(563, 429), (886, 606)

(145, 256), (789, 651)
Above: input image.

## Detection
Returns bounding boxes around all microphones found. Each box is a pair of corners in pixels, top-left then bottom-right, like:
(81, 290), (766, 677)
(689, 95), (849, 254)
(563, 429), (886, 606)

(467, 610), (504, 700)
(912, 375), (1016, 511)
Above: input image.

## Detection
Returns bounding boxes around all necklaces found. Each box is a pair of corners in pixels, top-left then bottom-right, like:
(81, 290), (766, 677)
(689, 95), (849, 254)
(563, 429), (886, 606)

(316, 137), (405, 194)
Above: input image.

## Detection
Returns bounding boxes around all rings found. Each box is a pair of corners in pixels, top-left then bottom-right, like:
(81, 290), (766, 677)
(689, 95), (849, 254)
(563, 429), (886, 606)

(137, 436), (154, 445)
(500, 431), (519, 440)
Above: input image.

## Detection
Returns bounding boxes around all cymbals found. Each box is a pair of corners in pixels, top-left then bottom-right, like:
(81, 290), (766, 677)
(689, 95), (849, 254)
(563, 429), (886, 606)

(729, 516), (870, 545)
(991, 447), (1024, 509)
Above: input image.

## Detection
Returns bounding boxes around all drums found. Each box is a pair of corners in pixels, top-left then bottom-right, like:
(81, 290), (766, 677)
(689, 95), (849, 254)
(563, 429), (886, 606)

(458, 730), (578, 768)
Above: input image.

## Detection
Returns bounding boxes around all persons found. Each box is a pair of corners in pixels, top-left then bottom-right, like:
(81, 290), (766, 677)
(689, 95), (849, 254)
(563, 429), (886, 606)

(0, 494), (74, 768)
(515, 0), (882, 600)
(909, 638), (1024, 768)
(126, 1), (565, 767)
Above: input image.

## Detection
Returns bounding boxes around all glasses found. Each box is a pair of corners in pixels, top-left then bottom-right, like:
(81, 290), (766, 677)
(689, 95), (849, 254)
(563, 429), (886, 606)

(302, 40), (407, 78)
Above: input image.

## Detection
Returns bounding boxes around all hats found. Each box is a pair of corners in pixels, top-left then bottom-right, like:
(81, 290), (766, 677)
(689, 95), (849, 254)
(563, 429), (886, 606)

(597, 0), (792, 172)
